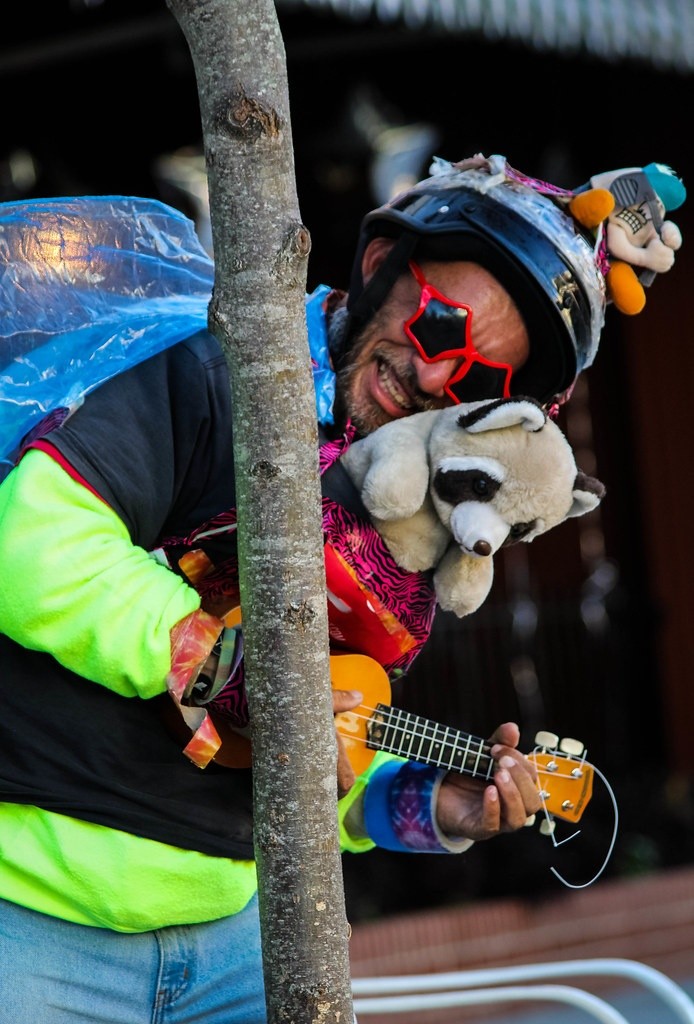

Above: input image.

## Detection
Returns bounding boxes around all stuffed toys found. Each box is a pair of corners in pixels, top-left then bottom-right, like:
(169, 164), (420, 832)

(148, 397), (609, 678)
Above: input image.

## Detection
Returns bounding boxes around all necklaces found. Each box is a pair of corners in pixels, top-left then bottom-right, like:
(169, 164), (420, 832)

(1, 153), (687, 1024)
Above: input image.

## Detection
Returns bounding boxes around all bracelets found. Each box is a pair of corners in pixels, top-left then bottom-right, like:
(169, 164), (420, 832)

(166, 612), (247, 767)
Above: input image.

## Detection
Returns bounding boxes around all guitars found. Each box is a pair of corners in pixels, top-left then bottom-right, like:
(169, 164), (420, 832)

(204, 599), (595, 827)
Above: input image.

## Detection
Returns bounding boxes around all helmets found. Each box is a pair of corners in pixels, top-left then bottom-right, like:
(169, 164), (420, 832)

(345, 152), (607, 405)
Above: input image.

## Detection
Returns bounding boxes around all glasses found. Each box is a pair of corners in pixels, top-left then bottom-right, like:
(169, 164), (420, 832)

(402, 261), (515, 404)
